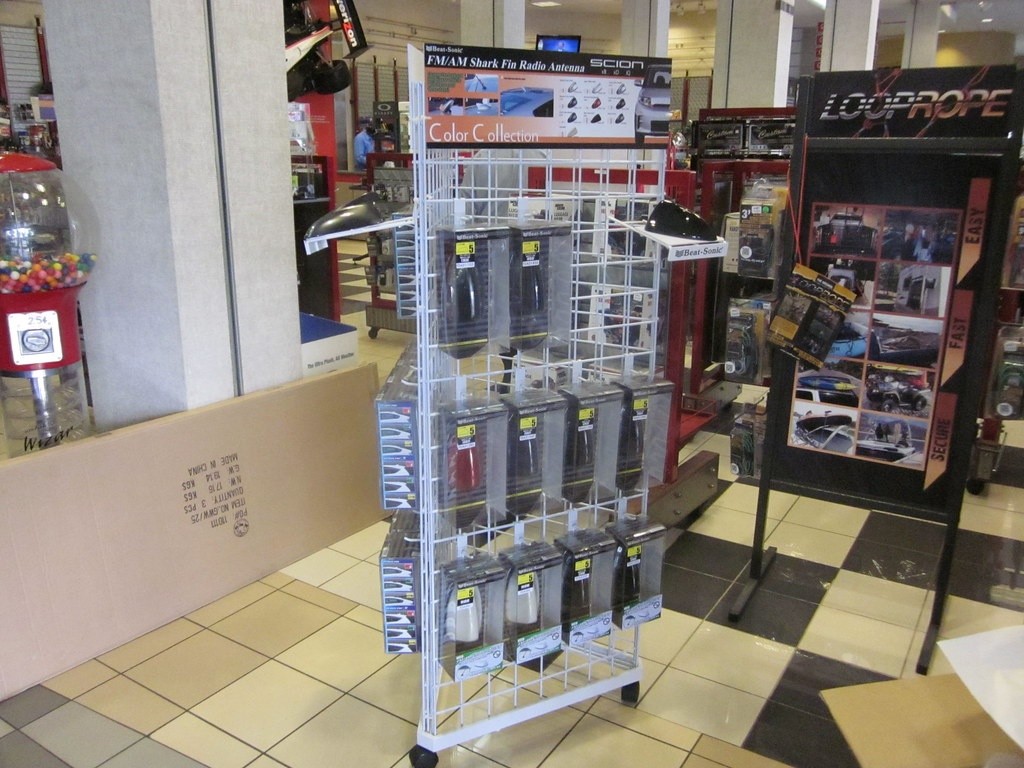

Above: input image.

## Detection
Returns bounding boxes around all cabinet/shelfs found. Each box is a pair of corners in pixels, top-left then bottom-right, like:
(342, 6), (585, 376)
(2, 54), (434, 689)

(305, 33), (1023, 767)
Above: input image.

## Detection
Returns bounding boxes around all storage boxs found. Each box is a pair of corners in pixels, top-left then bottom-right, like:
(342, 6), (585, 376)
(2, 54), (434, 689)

(1, 362), (397, 704)
(300, 313), (359, 375)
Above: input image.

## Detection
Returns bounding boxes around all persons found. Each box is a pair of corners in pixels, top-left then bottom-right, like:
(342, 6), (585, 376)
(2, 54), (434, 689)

(353, 126), (378, 170)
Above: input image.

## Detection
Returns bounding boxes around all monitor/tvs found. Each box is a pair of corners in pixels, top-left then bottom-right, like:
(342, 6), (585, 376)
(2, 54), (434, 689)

(536, 34), (581, 53)
